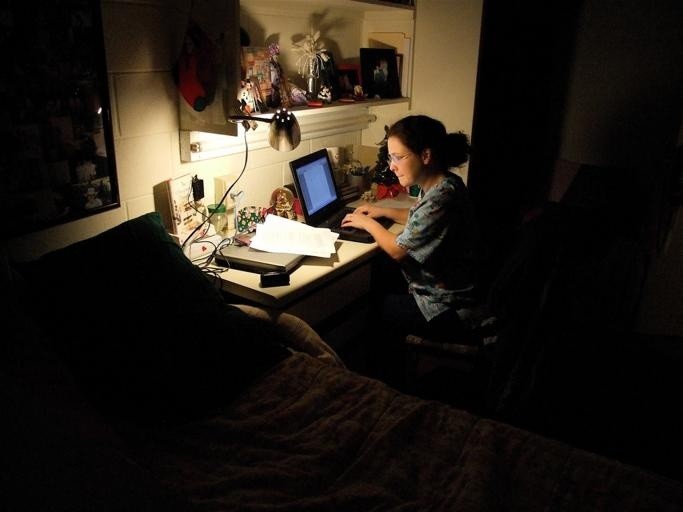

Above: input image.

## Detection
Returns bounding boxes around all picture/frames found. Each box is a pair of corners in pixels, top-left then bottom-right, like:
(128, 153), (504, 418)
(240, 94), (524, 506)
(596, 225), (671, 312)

(0, 0), (121, 233)
(396, 54), (404, 92)
(332, 64), (363, 99)
(360, 49), (402, 98)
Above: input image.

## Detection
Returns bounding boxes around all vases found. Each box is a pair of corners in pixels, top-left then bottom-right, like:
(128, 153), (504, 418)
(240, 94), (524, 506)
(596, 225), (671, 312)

(346, 175), (365, 188)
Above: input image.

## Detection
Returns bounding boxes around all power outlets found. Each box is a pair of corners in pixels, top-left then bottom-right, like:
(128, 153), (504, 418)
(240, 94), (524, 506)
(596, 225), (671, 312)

(214, 173), (237, 210)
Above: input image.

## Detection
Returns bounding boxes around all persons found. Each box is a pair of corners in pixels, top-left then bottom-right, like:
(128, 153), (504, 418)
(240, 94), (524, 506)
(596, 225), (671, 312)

(340, 114), (476, 371)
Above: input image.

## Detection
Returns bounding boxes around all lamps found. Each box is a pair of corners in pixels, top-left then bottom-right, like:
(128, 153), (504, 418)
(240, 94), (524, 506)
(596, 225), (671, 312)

(180, 107), (376, 163)
(228, 99), (300, 152)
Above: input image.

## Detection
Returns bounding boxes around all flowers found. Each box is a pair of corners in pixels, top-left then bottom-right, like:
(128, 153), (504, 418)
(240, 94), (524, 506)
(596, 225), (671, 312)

(332, 153), (376, 175)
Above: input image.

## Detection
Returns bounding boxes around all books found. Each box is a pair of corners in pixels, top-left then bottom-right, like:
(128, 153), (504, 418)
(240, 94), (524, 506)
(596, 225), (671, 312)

(167, 174), (204, 247)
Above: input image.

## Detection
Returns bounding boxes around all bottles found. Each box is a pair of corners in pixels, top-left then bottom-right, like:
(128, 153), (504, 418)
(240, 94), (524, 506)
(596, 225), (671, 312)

(207, 204), (229, 237)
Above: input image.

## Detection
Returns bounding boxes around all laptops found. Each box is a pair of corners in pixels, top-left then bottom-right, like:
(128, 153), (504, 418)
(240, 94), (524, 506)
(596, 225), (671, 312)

(288, 147), (395, 244)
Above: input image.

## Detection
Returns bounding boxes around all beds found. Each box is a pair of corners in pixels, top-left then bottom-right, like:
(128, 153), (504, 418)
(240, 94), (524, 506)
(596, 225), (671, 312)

(0, 337), (683, 512)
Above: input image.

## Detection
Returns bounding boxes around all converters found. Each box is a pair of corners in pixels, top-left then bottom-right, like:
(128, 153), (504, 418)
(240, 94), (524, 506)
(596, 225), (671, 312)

(191, 174), (204, 201)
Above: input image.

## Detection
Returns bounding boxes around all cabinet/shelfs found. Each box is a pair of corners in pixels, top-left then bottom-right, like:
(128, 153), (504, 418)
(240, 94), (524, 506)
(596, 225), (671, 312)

(178, 0), (417, 137)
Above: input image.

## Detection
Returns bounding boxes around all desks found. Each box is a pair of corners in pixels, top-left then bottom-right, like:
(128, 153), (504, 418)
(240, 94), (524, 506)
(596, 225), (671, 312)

(190, 192), (437, 307)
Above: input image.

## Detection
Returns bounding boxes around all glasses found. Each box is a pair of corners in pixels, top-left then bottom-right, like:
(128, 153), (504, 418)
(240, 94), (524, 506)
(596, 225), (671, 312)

(387, 152), (413, 164)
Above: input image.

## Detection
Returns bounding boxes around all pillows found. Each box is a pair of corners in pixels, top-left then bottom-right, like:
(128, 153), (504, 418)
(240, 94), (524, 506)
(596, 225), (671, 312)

(9, 215), (266, 431)
